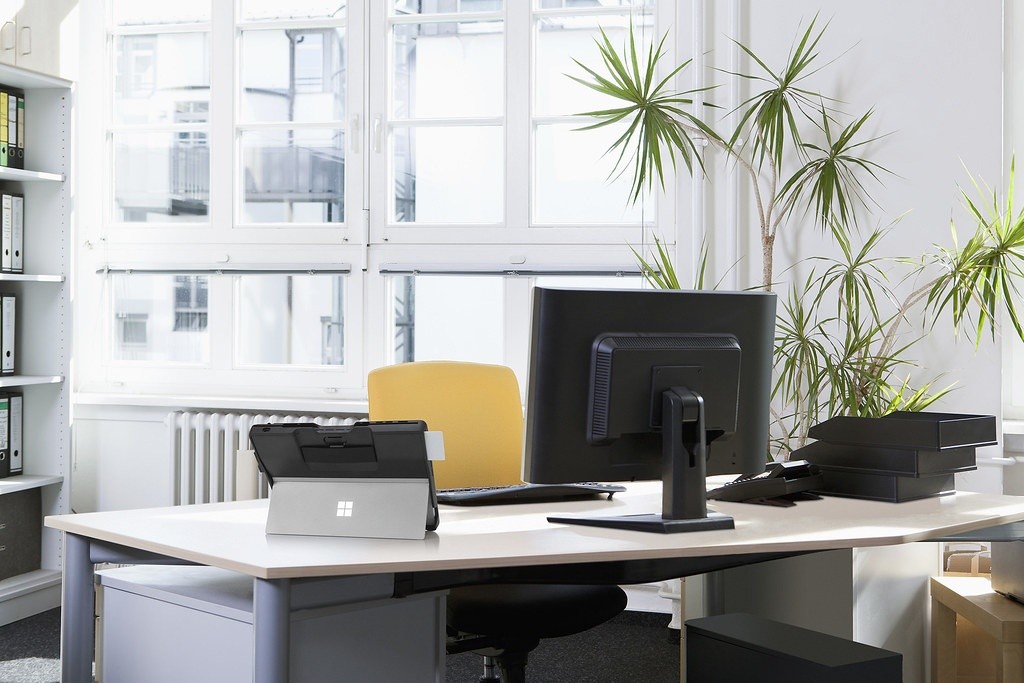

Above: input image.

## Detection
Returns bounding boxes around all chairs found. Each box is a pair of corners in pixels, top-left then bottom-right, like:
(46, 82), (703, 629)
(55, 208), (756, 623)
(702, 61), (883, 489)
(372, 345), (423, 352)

(366, 357), (629, 681)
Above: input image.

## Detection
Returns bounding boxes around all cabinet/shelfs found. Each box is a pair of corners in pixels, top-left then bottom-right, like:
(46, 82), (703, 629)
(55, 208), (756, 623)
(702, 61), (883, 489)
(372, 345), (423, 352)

(0, 56), (69, 628)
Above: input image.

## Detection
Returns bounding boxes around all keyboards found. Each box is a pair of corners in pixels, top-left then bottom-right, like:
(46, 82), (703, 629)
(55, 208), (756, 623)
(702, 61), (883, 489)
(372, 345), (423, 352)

(434, 483), (615, 506)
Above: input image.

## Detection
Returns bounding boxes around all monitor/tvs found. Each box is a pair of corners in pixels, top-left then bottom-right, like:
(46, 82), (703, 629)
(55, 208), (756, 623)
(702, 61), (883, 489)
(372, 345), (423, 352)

(249, 419), (439, 531)
(522, 285), (777, 485)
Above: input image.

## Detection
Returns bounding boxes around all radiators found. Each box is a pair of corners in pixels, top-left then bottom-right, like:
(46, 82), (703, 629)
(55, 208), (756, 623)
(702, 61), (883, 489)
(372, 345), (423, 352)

(158, 412), (369, 507)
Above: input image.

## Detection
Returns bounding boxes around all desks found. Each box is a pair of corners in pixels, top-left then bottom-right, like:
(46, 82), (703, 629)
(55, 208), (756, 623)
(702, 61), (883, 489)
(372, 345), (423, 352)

(41, 471), (1024, 682)
